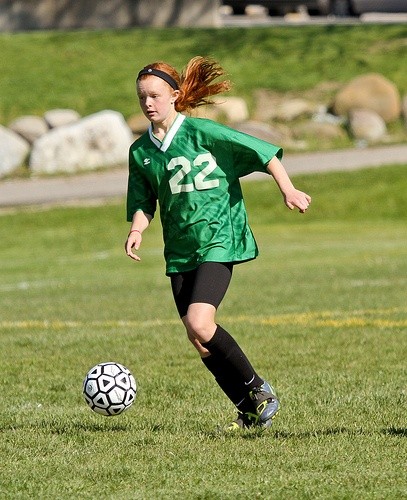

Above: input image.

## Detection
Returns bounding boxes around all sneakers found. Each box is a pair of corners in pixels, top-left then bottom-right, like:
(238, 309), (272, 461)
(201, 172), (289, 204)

(249, 382), (280, 421)
(224, 411), (272, 436)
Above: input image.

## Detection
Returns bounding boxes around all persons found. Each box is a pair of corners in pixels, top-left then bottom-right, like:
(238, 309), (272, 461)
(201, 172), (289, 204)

(125, 55), (311, 432)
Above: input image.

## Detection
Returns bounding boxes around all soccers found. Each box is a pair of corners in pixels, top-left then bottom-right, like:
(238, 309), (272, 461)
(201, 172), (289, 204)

(84, 362), (138, 416)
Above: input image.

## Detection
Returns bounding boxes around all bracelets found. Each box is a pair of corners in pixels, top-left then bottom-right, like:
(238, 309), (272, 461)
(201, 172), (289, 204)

(128, 230), (140, 237)
